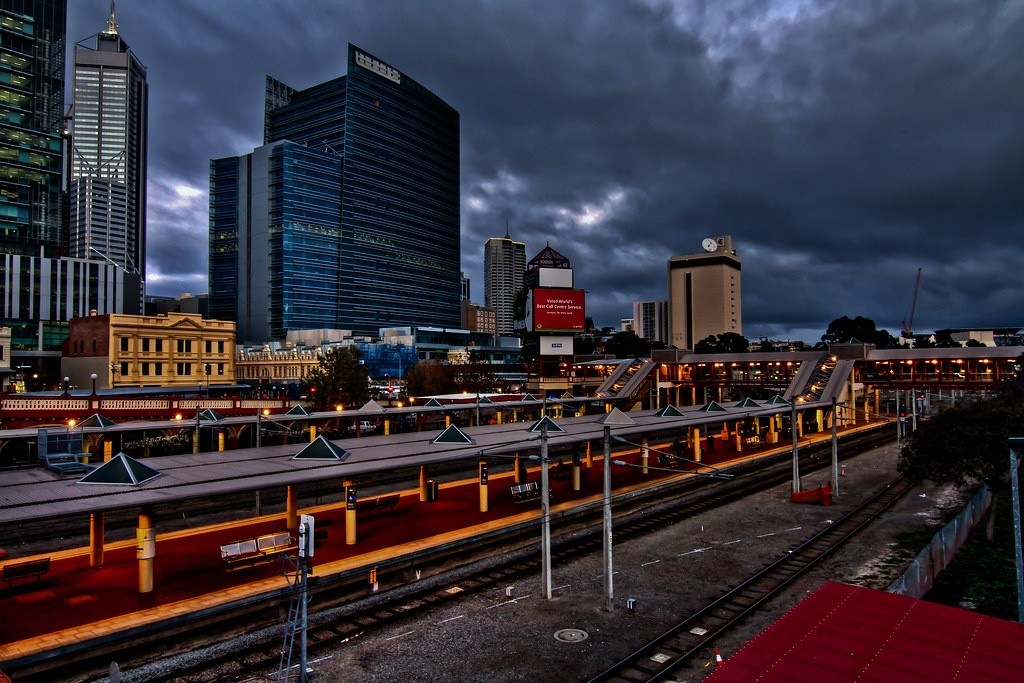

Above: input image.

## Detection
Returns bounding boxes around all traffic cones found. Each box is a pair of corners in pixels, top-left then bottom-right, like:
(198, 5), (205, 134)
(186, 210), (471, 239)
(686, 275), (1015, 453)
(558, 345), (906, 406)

(714, 647), (726, 669)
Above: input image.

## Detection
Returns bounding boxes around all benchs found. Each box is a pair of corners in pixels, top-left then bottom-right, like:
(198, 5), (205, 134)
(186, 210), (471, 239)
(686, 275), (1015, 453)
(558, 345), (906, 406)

(509, 481), (554, 507)
(746, 435), (764, 449)
(656, 454), (679, 468)
(219, 531), (300, 575)
(2, 558), (51, 590)
(356, 494), (400, 520)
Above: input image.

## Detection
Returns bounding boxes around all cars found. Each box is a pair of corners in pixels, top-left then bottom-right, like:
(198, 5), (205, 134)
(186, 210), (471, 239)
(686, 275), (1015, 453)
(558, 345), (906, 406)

(347, 421), (377, 434)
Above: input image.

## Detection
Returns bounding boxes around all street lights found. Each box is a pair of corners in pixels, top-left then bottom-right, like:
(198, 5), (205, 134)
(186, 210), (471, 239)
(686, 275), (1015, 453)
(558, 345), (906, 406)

(90, 374), (98, 396)
(64, 377), (70, 394)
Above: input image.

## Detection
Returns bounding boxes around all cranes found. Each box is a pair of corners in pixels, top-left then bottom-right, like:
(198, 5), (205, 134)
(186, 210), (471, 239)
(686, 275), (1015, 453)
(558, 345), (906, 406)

(901, 267), (922, 339)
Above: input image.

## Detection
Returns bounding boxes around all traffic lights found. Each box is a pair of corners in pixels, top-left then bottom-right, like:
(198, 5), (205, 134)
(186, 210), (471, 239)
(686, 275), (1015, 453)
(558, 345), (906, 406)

(900, 411), (907, 417)
